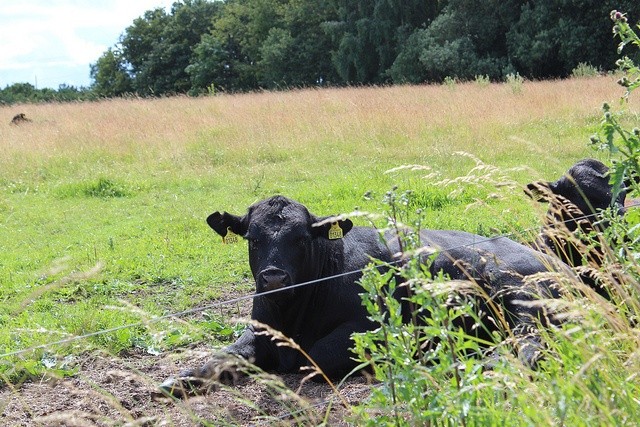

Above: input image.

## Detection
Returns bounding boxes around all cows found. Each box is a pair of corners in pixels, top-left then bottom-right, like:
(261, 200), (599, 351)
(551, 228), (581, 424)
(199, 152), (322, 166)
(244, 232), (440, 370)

(526, 156), (638, 328)
(147, 192), (587, 398)
